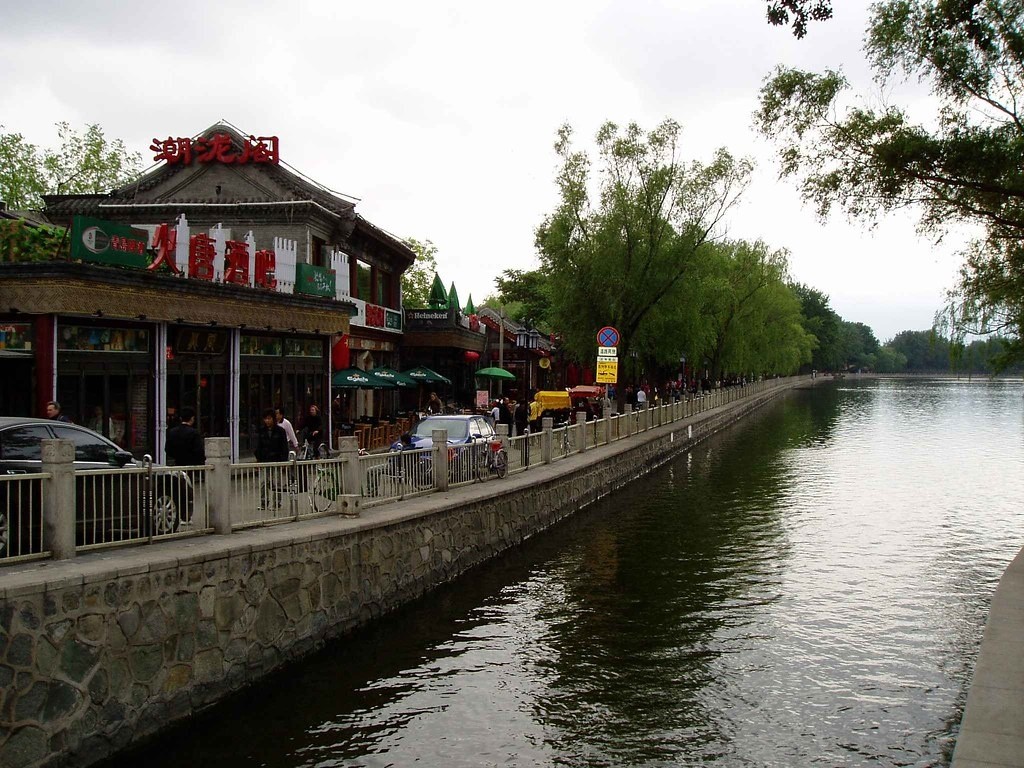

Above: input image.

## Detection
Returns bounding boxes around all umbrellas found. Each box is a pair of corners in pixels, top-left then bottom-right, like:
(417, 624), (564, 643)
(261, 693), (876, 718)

(427, 274), (476, 315)
(329, 365), (516, 427)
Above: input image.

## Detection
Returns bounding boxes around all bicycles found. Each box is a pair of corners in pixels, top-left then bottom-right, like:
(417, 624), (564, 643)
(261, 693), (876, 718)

(410, 439), (470, 491)
(311, 447), (371, 510)
(475, 433), (510, 483)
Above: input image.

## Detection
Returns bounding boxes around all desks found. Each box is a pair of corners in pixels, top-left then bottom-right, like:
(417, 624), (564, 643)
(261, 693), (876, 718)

(396, 418), (408, 439)
(379, 421), (389, 445)
(355, 424), (372, 450)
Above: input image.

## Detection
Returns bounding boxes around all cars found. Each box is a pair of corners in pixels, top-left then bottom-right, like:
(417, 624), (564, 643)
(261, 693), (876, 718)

(0, 416), (194, 558)
(387, 415), (498, 485)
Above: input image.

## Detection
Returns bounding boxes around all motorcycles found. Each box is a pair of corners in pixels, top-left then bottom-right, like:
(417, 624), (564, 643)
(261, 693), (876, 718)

(537, 384), (603, 429)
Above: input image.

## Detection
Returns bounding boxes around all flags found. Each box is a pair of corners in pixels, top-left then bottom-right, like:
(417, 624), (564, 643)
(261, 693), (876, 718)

(333, 336), (350, 370)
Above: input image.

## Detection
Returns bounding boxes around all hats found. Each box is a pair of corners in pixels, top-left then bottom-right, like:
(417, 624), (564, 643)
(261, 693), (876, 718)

(491, 402), (498, 407)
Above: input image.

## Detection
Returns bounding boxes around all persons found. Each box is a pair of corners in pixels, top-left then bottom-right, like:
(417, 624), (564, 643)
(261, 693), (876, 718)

(570, 376), (760, 415)
(46, 392), (545, 512)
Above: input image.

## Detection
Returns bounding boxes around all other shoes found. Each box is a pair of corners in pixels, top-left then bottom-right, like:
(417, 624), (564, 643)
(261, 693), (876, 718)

(269, 507), (280, 510)
(180, 520), (193, 525)
(364, 487), (378, 497)
(257, 507), (268, 511)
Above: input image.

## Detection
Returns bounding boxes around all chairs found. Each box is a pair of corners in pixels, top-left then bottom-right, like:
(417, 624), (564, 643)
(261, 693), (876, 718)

(333, 413), (419, 451)
